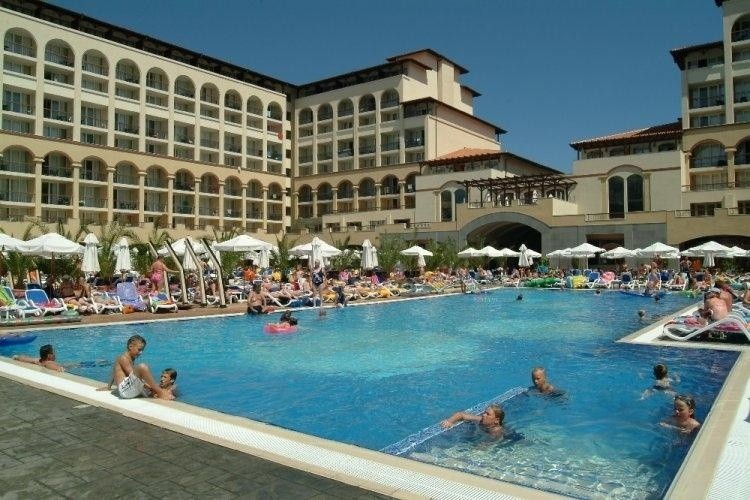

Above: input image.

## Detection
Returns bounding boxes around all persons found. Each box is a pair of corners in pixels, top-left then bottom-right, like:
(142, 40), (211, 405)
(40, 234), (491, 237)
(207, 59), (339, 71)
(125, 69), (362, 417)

(12, 254), (750, 444)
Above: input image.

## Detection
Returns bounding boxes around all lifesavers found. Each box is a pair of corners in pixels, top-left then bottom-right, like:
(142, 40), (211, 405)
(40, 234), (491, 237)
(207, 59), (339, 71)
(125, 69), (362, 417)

(262, 324), (297, 335)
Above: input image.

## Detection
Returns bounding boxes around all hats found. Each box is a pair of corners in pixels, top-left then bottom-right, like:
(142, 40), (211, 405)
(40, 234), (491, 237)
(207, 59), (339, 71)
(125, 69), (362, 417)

(710, 287), (722, 294)
